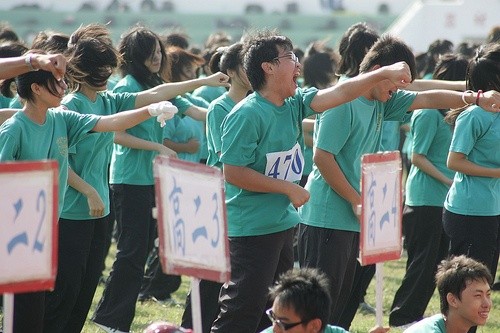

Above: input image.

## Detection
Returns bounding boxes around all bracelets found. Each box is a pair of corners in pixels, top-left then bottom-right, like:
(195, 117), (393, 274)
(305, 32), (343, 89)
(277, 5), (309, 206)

(24, 52), (39, 71)
(462, 88), (475, 105)
(475, 88), (483, 106)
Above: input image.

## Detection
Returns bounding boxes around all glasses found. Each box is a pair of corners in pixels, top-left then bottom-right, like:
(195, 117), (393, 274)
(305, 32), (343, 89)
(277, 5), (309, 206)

(266, 307), (308, 332)
(272, 53), (299, 65)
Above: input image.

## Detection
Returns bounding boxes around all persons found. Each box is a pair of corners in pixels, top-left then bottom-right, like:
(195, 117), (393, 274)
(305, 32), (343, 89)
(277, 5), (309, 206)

(209, 28), (411, 333)
(298, 36), (500, 332)
(440, 41), (500, 296)
(47, 27), (233, 332)
(88, 22), (209, 331)
(400, 254), (495, 333)
(1, 24), (500, 234)
(388, 52), (473, 326)
(256, 267), (352, 333)
(0, 48), (180, 332)
(135, 45), (211, 310)
(181, 40), (318, 332)
